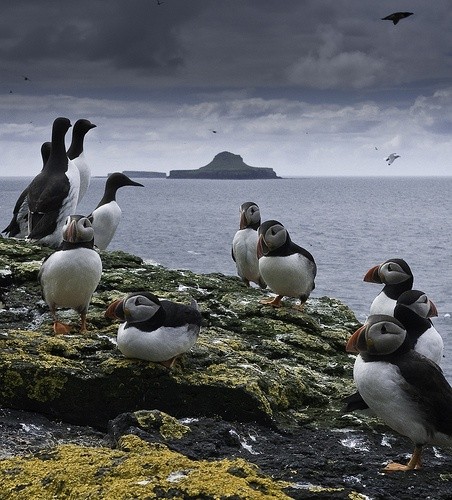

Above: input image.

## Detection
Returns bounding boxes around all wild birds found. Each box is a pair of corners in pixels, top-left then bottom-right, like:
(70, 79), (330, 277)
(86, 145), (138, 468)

(232, 201), (317, 312)
(208, 128), (218, 134)
(381, 12), (415, 27)
(346, 258), (452, 476)
(104, 291), (203, 370)
(3, 116), (145, 334)
(385, 152), (400, 167)
(21, 75), (31, 82)
